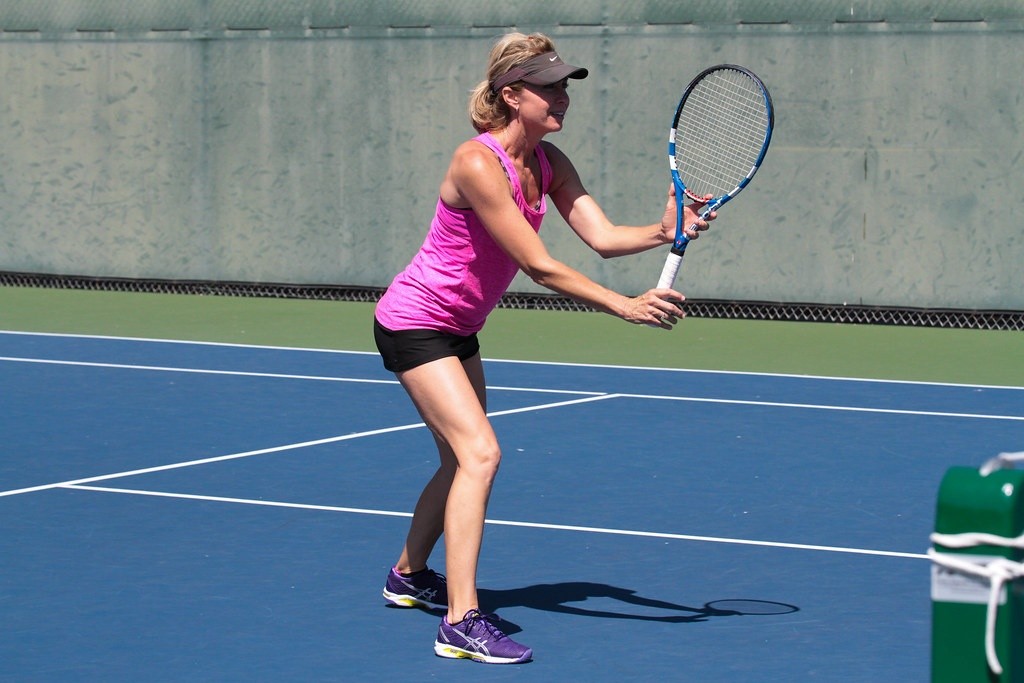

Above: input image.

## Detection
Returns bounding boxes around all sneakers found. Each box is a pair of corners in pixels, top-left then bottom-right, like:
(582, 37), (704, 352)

(382, 564), (451, 611)
(435, 607), (532, 664)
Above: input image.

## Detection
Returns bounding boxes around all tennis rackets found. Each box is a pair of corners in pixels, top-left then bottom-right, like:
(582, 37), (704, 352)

(646, 63), (775, 328)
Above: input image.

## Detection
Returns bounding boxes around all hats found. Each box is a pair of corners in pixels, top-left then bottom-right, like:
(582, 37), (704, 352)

(491, 52), (588, 96)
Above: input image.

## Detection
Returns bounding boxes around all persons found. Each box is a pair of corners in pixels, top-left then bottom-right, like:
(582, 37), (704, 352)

(372, 33), (719, 663)
